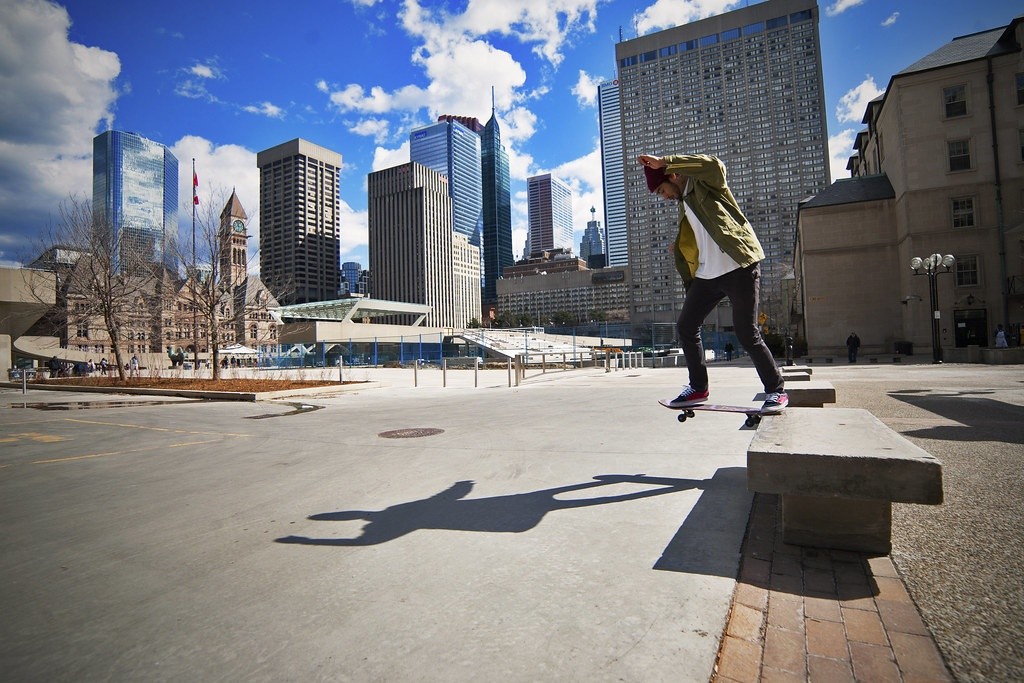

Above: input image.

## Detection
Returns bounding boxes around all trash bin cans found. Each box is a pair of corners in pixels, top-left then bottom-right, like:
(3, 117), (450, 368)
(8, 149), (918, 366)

(893, 341), (914, 356)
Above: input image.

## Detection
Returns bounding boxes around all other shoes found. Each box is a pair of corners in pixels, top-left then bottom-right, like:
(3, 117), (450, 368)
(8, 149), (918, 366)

(848, 360), (853, 363)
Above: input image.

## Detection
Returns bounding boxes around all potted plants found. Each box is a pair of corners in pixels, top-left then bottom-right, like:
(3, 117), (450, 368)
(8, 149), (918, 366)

(34, 367), (51, 380)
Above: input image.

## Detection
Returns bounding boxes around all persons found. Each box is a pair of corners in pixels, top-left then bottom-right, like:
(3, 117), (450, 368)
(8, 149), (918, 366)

(167, 347), (175, 366)
(126, 363), (129, 369)
(635, 153), (789, 413)
(100, 358), (108, 375)
(187, 346), (191, 352)
(12, 365), (20, 382)
(992, 324), (1008, 348)
(131, 353), (138, 377)
(846, 332), (860, 363)
(89, 359), (94, 372)
(223, 356), (228, 369)
(231, 356), (236, 367)
(72, 363), (82, 376)
(49, 356), (58, 378)
(725, 341), (734, 361)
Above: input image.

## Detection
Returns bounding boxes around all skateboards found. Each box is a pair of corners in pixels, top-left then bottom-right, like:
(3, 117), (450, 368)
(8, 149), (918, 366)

(658, 398), (785, 427)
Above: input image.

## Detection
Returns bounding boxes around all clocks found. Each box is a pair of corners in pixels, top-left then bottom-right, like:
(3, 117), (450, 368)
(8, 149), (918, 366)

(233, 219), (244, 233)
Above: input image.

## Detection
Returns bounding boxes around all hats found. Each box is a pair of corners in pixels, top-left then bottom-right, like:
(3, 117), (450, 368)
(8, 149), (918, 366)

(644, 165), (672, 193)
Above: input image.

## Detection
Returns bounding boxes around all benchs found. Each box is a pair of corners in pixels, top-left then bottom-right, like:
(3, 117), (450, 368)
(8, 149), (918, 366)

(782, 365), (812, 375)
(783, 380), (838, 407)
(782, 371), (810, 380)
(741, 405), (946, 550)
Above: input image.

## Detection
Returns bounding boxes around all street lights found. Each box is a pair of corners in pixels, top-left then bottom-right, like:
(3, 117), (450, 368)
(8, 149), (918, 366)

(910, 254), (956, 364)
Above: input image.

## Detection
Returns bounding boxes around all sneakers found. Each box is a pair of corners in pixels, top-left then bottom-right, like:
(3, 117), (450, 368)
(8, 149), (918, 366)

(760, 392), (789, 413)
(669, 384), (709, 406)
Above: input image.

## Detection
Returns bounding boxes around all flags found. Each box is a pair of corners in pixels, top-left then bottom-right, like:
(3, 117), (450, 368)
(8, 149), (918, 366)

(758, 311), (768, 325)
(763, 327), (768, 334)
(194, 172), (198, 186)
(194, 189), (199, 205)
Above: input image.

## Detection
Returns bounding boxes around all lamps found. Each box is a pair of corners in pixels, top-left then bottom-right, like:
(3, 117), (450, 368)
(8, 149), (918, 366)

(966, 292), (975, 305)
(899, 299), (907, 305)
(906, 295), (922, 302)
